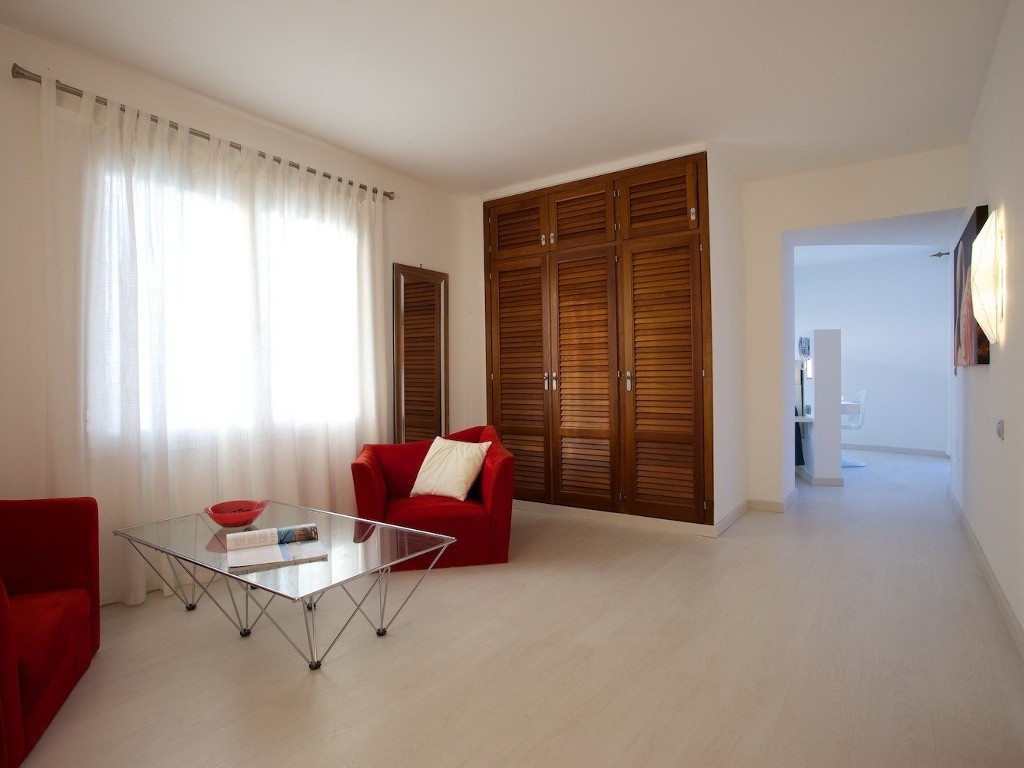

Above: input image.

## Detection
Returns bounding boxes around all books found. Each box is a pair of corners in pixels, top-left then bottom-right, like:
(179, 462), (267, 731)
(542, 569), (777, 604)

(226, 523), (328, 573)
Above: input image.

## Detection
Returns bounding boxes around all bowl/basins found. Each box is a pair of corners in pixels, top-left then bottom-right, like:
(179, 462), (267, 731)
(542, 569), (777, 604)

(203, 500), (267, 527)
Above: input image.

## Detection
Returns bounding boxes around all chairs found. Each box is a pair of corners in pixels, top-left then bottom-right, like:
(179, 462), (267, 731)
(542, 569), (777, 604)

(840, 390), (867, 467)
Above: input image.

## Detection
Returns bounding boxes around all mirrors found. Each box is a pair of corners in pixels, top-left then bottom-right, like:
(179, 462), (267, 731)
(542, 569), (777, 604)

(392, 262), (450, 445)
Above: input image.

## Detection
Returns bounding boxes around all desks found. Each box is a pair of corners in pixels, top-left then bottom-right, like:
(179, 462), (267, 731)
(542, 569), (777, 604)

(113, 500), (456, 670)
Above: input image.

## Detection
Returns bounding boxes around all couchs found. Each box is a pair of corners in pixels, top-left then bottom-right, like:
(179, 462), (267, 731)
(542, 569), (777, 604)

(0, 497), (102, 768)
(349, 426), (516, 573)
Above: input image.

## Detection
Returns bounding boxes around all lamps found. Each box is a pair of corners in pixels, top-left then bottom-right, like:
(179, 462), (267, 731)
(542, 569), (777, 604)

(969, 210), (1005, 344)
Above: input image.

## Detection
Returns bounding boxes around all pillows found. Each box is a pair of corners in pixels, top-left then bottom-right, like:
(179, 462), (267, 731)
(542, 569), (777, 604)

(409, 435), (492, 502)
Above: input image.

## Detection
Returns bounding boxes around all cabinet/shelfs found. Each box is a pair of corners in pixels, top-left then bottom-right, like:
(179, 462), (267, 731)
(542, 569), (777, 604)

(480, 151), (714, 525)
